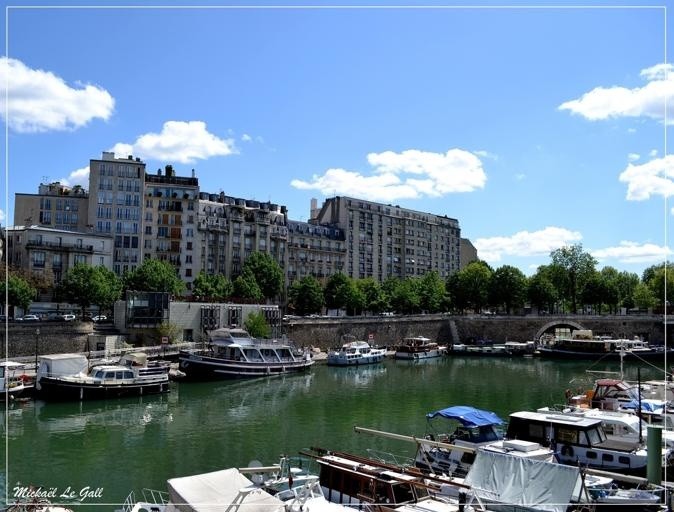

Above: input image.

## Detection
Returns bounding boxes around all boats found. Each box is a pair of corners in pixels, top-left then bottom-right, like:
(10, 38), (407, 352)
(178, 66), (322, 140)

(5, 378), (674, 512)
(0, 324), (674, 400)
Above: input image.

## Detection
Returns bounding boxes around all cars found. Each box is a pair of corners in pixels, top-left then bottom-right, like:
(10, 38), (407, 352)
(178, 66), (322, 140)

(91, 315), (107, 322)
(14, 314), (40, 320)
(62, 315), (75, 321)
